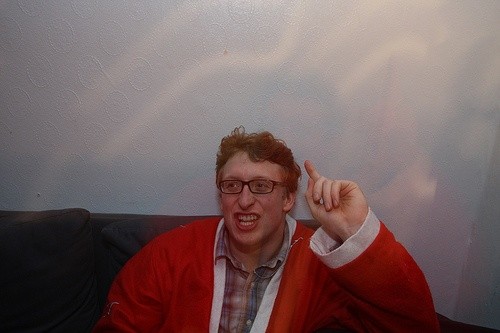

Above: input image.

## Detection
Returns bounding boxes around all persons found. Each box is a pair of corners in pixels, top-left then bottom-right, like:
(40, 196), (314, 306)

(90, 131), (441, 333)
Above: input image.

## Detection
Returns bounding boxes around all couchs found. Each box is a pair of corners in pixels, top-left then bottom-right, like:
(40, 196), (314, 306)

(0, 207), (323, 333)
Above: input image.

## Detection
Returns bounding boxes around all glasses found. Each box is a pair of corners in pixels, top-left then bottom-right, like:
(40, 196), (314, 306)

(217, 179), (289, 194)
